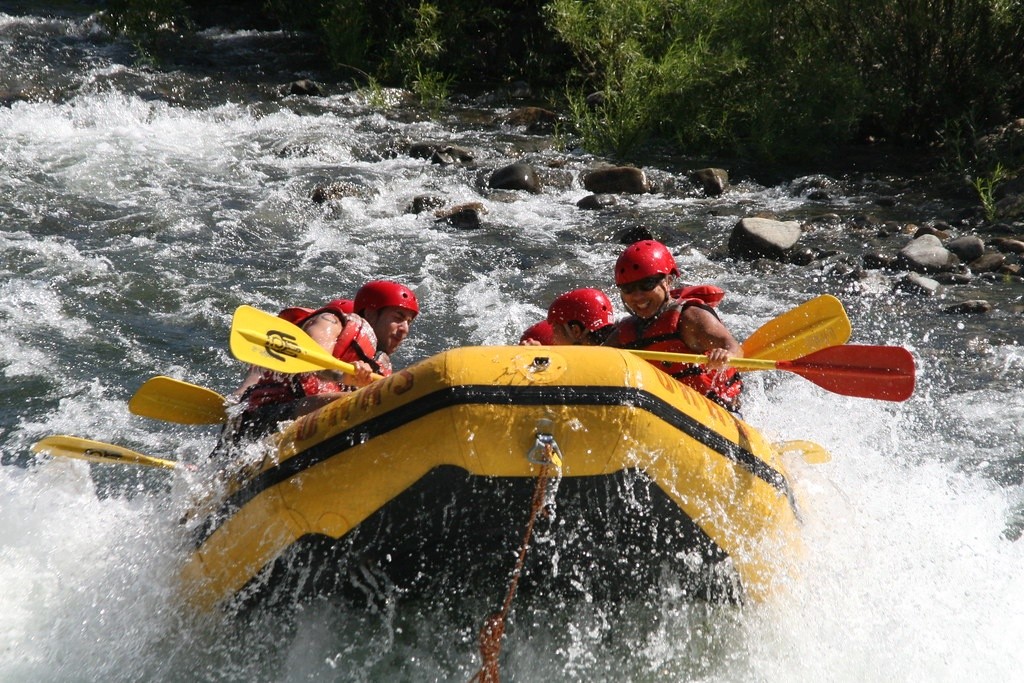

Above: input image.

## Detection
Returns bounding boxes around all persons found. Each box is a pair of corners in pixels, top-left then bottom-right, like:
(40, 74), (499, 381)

(518, 240), (745, 423)
(210, 280), (420, 448)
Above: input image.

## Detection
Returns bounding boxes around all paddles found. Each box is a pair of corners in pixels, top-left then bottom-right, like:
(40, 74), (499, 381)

(230, 304), (385, 378)
(616, 341), (914, 402)
(36, 437), (199, 476)
(698, 293), (851, 392)
(134, 374), (254, 426)
(775, 438), (827, 467)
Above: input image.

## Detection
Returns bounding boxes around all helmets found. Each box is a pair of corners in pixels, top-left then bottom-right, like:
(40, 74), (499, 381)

(352, 281), (418, 321)
(615, 240), (680, 288)
(323, 299), (354, 314)
(547, 289), (614, 331)
(518, 319), (556, 346)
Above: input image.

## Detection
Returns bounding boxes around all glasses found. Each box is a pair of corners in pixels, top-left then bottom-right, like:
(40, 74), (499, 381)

(619, 274), (665, 294)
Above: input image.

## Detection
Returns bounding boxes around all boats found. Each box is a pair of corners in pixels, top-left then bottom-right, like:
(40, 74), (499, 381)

(173, 345), (834, 629)
